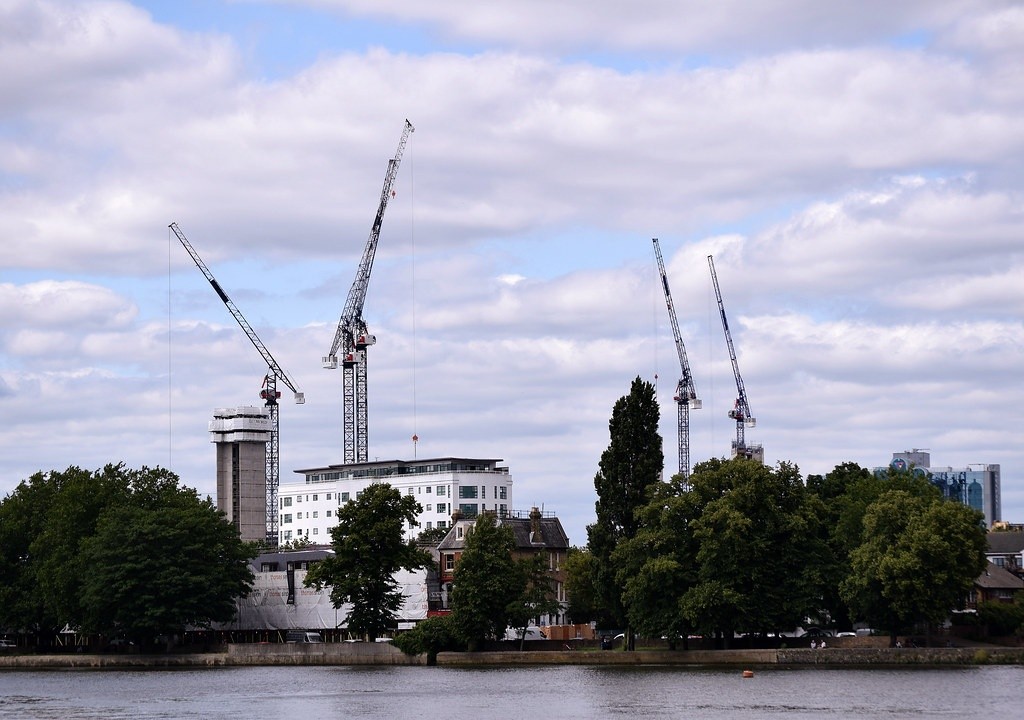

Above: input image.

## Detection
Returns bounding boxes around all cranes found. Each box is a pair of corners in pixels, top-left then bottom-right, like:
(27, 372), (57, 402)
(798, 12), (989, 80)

(164, 222), (304, 551)
(649, 239), (703, 495)
(705, 254), (762, 467)
(323, 117), (425, 465)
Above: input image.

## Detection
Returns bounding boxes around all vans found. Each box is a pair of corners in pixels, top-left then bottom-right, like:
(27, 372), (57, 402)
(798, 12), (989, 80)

(503, 624), (546, 640)
(302, 632), (324, 644)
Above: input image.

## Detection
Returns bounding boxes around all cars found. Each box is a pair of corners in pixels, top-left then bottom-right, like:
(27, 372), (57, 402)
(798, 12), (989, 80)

(801, 630), (857, 637)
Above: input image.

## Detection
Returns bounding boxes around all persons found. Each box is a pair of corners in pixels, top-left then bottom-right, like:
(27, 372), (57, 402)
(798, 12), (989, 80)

(895, 640), (903, 649)
(820, 639), (828, 649)
(811, 640), (817, 649)
